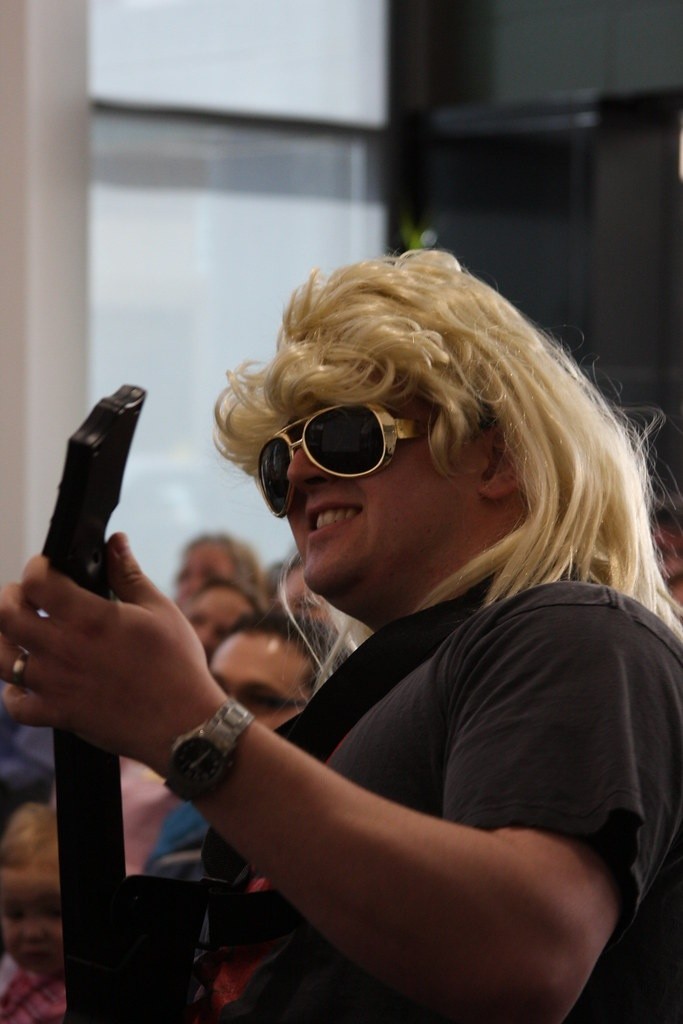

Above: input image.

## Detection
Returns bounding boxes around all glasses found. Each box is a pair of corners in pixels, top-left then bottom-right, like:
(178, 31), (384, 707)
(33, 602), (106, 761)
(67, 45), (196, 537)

(258, 402), (434, 519)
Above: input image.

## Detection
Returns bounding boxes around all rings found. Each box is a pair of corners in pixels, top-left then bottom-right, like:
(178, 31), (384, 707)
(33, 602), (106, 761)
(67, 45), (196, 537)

(11, 649), (31, 687)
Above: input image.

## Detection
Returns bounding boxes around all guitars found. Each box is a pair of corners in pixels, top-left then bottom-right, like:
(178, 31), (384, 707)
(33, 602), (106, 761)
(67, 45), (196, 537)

(44, 384), (217, 1021)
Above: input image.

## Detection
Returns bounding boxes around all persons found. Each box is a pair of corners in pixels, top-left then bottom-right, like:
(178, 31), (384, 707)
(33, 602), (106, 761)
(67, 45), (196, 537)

(0, 248), (683, 1024)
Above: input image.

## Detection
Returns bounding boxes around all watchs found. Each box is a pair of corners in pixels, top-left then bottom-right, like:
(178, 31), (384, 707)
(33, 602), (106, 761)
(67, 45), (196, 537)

(166, 694), (255, 805)
